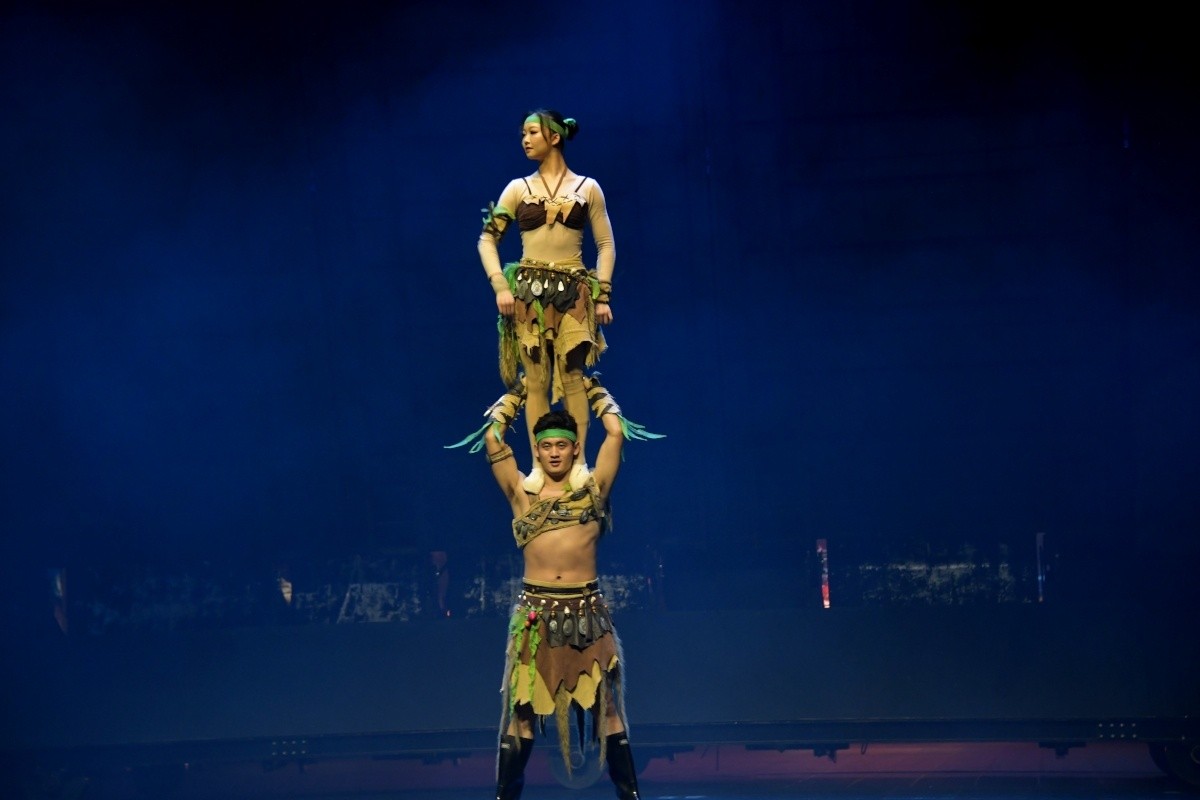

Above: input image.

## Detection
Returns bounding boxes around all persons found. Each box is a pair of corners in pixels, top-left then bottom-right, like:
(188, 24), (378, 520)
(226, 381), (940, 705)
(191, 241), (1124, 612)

(483, 374), (641, 800)
(477, 106), (618, 495)
